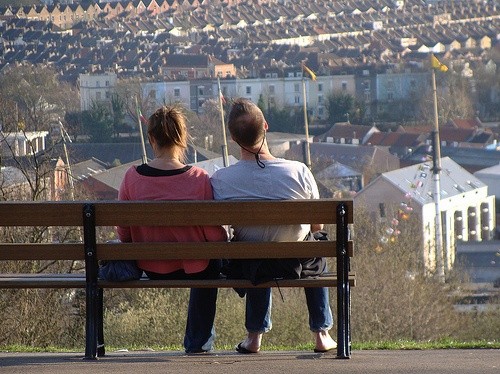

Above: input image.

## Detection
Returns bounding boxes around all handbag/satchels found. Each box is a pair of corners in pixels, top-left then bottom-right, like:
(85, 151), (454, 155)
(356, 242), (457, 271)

(94, 238), (142, 282)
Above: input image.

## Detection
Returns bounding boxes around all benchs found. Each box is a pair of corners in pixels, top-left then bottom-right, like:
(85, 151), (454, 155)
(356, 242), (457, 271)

(0, 200), (357, 361)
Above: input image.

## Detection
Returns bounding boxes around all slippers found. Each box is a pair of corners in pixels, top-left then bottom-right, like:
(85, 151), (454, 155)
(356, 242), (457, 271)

(235, 342), (257, 353)
(314, 342), (338, 353)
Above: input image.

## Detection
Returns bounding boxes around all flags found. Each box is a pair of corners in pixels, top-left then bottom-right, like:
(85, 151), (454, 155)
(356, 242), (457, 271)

(432, 54), (448, 72)
(221, 92), (226, 106)
(302, 64), (316, 81)
(138, 107), (147, 123)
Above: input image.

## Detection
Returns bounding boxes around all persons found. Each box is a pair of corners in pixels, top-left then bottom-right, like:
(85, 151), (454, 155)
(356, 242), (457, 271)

(210, 97), (337, 353)
(117, 106), (230, 353)
